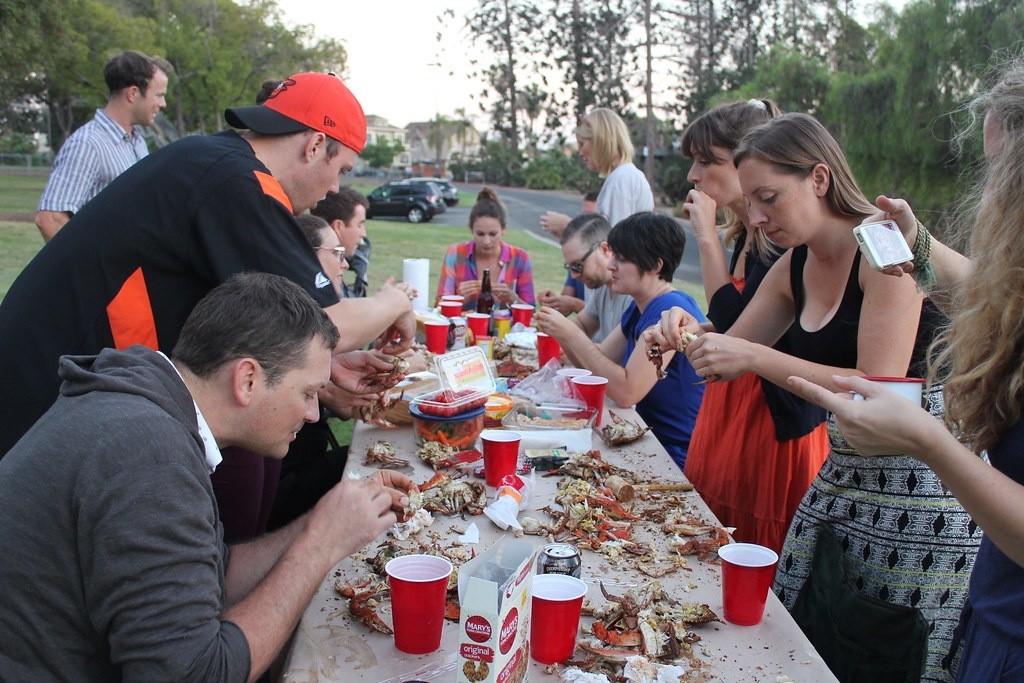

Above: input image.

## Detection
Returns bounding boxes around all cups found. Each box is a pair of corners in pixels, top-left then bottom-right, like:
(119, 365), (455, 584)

(442, 295), (464, 304)
(493, 316), (510, 340)
(571, 376), (608, 426)
(439, 301), (464, 318)
(718, 543), (778, 626)
(511, 303), (534, 327)
(466, 313), (491, 346)
(529, 574), (588, 665)
(424, 321), (450, 354)
(556, 368), (593, 419)
(385, 554), (453, 654)
(476, 335), (492, 362)
(479, 430), (522, 487)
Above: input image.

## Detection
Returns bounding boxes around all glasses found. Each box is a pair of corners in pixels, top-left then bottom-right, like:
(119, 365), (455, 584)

(315, 245), (346, 265)
(564, 239), (608, 276)
(576, 114), (590, 128)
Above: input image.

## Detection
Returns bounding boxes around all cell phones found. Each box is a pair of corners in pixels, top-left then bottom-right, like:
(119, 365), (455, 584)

(853, 219), (914, 271)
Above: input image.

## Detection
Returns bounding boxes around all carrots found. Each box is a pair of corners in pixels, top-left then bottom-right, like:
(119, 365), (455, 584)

(415, 416), (483, 450)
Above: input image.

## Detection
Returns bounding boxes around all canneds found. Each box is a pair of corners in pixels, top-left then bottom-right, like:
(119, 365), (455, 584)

(537, 543), (582, 580)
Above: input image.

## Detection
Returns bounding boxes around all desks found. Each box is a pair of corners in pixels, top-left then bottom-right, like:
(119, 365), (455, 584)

(284, 336), (841, 683)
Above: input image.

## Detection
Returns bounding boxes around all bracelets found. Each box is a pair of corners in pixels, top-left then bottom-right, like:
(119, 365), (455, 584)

(911, 218), (937, 290)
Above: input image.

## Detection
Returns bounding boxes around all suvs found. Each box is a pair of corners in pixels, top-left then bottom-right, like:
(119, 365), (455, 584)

(400, 175), (460, 207)
(361, 180), (448, 224)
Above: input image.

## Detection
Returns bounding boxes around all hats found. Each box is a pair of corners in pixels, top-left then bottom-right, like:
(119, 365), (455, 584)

(223, 72), (366, 154)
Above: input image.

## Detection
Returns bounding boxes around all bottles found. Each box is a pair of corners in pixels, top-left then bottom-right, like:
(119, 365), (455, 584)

(476, 268), (494, 337)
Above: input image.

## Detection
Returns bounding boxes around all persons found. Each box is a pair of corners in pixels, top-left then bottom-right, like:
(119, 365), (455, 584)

(213, 188), (369, 541)
(1, 274), (421, 683)
(682, 98), (830, 584)
(644, 113), (990, 683)
(788, 67), (1024, 683)
(556, 212), (634, 363)
(537, 191), (600, 317)
(36, 50), (172, 244)
(540, 108), (655, 305)
(0, 73), (418, 456)
(533, 212), (708, 472)
(435, 188), (536, 314)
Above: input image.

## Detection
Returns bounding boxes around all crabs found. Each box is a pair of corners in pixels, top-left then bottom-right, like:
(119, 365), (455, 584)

(597, 409), (652, 446)
(679, 329), (723, 386)
(493, 338), (512, 361)
(358, 352), (411, 387)
(333, 434), (735, 683)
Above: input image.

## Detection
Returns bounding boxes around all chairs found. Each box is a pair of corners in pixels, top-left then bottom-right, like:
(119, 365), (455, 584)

(212, 406), (349, 546)
(792, 524), (928, 683)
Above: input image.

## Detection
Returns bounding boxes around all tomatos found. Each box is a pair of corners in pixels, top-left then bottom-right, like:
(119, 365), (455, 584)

(418, 390), (488, 416)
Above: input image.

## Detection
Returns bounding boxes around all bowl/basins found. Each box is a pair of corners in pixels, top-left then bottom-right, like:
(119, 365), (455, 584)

(484, 394), (513, 428)
(505, 331), (548, 371)
(413, 307), (450, 344)
(407, 402), (485, 451)
(501, 402), (599, 438)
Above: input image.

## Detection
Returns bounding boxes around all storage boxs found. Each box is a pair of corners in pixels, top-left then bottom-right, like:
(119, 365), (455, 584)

(457, 534), (539, 682)
